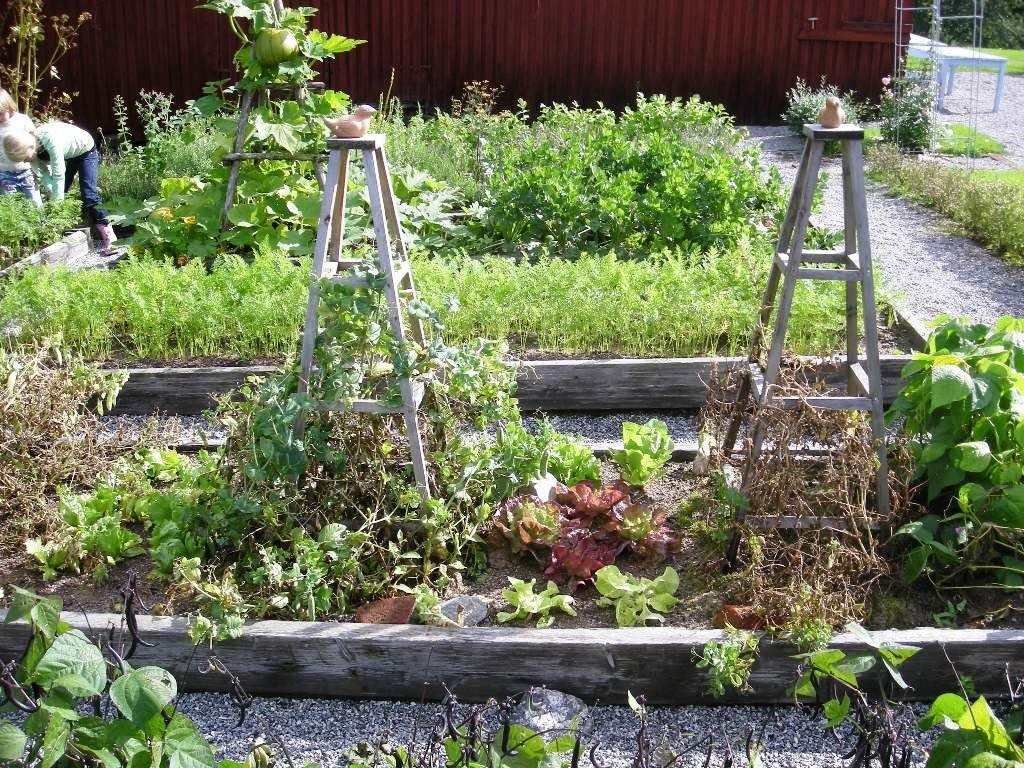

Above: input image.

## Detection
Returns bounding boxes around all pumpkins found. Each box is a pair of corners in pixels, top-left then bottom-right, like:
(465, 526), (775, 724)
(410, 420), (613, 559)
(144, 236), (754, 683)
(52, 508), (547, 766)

(255, 28), (300, 63)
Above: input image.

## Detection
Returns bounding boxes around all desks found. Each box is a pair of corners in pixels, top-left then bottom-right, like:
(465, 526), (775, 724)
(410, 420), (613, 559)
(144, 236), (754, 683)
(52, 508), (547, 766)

(909, 45), (1009, 115)
(908, 33), (949, 92)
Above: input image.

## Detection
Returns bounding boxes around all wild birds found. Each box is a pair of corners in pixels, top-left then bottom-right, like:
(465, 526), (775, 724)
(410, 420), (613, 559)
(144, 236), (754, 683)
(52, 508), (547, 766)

(817, 97), (848, 128)
(324, 105), (378, 139)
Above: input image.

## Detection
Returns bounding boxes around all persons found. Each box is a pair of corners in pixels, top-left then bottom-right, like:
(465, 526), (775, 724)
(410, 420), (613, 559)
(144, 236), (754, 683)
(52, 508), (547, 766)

(0, 88), (42, 208)
(3, 123), (119, 256)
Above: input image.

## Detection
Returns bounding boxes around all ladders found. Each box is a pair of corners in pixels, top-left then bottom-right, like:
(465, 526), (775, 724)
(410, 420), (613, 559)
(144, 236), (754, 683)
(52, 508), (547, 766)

(722, 123), (898, 572)
(286, 135), (451, 502)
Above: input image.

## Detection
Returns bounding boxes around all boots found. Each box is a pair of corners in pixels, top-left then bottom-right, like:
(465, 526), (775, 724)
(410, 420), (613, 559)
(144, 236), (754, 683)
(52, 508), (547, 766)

(97, 222), (117, 254)
